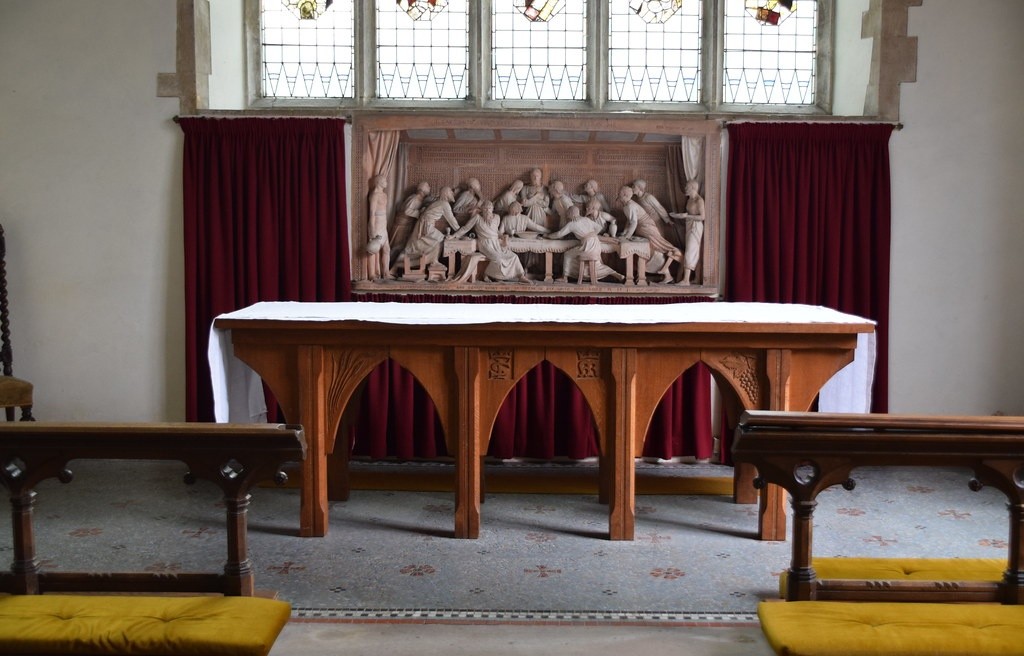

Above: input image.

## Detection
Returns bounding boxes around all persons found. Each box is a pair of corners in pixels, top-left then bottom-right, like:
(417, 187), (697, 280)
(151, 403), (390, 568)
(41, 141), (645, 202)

(367, 168), (705, 286)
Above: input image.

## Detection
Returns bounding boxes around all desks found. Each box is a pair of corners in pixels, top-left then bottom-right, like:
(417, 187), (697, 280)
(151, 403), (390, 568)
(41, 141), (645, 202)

(212, 301), (876, 540)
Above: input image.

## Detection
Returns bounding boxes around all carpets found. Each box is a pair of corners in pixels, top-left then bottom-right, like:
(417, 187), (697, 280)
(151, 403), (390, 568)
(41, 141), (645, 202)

(0, 443), (1013, 625)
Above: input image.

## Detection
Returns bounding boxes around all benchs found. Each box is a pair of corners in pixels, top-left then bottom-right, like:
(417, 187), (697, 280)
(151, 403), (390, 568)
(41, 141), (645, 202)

(757, 593), (1024, 656)
(780, 555), (1007, 597)
(0, 593), (290, 655)
